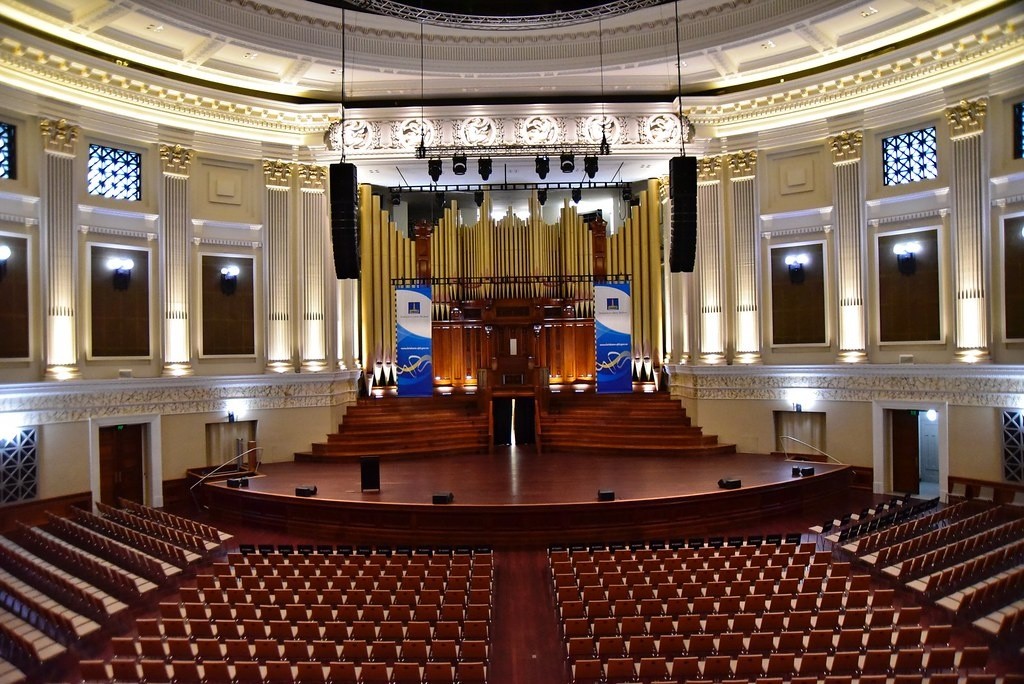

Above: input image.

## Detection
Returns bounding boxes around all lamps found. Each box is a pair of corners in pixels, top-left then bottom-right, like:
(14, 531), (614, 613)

(392, 187), (633, 207)
(428, 153), (599, 182)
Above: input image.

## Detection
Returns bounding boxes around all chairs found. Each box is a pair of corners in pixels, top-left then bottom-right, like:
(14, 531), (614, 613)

(0, 498), (1024, 684)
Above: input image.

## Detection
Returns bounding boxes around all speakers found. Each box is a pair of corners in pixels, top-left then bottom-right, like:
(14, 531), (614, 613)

(295, 485), (317, 497)
(792, 466), (814, 476)
(598, 489), (614, 499)
(433, 491), (454, 503)
(227, 477), (248, 488)
(718, 478), (741, 488)
(329, 163), (360, 279)
(669, 156), (697, 273)
